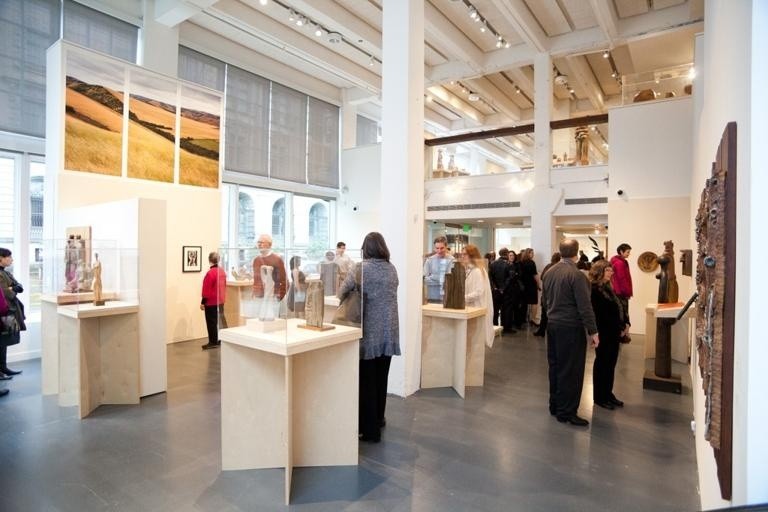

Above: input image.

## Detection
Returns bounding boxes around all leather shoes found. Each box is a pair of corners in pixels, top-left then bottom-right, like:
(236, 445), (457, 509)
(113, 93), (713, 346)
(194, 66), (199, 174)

(567, 415), (590, 427)
(1, 368), (23, 397)
(593, 396), (624, 410)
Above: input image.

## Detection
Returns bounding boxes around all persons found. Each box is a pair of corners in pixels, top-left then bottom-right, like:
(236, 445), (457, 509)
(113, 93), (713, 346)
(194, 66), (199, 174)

(423, 235), (605, 349)
(199, 252), (228, 349)
(336, 231), (401, 443)
(589, 259), (631, 410)
(609, 242), (634, 344)
(0, 247), (26, 397)
(316, 242), (354, 297)
(542, 238), (602, 427)
(254, 233), (291, 321)
(286, 256), (308, 319)
(68, 251), (103, 301)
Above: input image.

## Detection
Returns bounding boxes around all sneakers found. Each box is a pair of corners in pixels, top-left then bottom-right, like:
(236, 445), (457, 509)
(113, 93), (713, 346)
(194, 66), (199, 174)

(201, 344), (218, 350)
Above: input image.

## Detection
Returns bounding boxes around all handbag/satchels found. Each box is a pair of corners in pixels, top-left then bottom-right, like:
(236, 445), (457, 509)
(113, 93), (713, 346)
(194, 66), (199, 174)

(331, 289), (364, 328)
(2, 312), (20, 347)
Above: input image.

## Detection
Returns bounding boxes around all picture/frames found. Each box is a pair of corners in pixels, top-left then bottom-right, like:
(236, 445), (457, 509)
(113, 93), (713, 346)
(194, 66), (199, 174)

(182, 246), (202, 272)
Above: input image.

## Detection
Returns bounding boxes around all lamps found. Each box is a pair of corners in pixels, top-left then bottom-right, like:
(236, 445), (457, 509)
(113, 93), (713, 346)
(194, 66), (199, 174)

(288, 6), (374, 66)
(466, 4), (509, 48)
